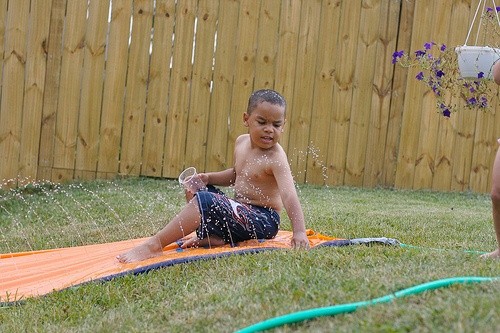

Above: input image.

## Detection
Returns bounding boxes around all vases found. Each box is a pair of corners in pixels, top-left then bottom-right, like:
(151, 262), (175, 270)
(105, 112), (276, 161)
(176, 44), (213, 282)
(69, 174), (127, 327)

(455, 46), (500, 80)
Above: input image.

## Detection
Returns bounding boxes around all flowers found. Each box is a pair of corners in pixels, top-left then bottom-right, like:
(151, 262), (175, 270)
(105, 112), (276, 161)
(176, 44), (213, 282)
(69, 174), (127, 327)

(392, 7), (500, 117)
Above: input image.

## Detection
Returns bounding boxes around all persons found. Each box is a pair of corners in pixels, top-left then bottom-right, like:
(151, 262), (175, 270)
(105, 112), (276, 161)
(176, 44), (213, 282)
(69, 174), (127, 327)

(116, 88), (311, 263)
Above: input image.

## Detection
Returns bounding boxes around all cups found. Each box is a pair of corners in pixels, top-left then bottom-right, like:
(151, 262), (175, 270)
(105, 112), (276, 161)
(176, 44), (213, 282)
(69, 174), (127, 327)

(178, 167), (208, 195)
